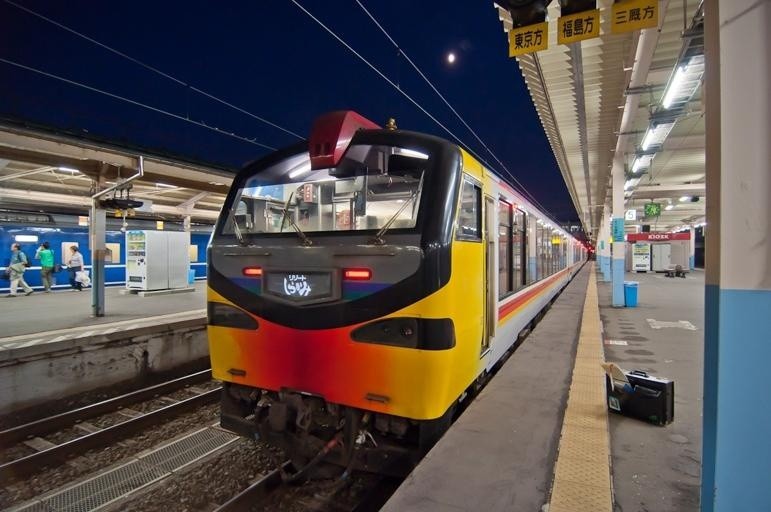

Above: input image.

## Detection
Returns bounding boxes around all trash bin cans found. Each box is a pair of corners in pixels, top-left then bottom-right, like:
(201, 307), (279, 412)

(624, 281), (638, 306)
(188, 270), (195, 283)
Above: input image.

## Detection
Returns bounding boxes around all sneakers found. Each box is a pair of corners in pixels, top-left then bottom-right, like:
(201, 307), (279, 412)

(45, 288), (51, 293)
(5, 294), (16, 297)
(25, 291), (33, 296)
(72, 289), (80, 292)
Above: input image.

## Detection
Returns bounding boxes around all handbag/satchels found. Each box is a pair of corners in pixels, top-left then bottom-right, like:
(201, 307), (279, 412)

(52, 264), (63, 273)
(75, 270), (90, 287)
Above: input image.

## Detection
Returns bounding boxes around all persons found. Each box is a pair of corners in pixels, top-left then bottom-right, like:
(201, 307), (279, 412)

(5, 242), (84, 297)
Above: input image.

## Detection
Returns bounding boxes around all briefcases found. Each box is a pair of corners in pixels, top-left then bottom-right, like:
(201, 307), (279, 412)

(605, 369), (674, 427)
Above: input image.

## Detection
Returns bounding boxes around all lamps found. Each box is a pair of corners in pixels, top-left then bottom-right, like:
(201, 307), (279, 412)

(621, 0), (705, 206)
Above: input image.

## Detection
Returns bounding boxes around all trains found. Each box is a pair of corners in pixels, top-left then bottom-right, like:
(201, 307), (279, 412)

(0, 224), (212, 293)
(205, 107), (588, 488)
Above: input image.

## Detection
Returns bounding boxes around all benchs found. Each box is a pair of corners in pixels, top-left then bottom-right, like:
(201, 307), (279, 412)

(664, 268), (690, 277)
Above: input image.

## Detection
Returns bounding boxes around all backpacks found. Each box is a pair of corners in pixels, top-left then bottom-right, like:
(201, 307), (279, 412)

(25, 256), (30, 267)
